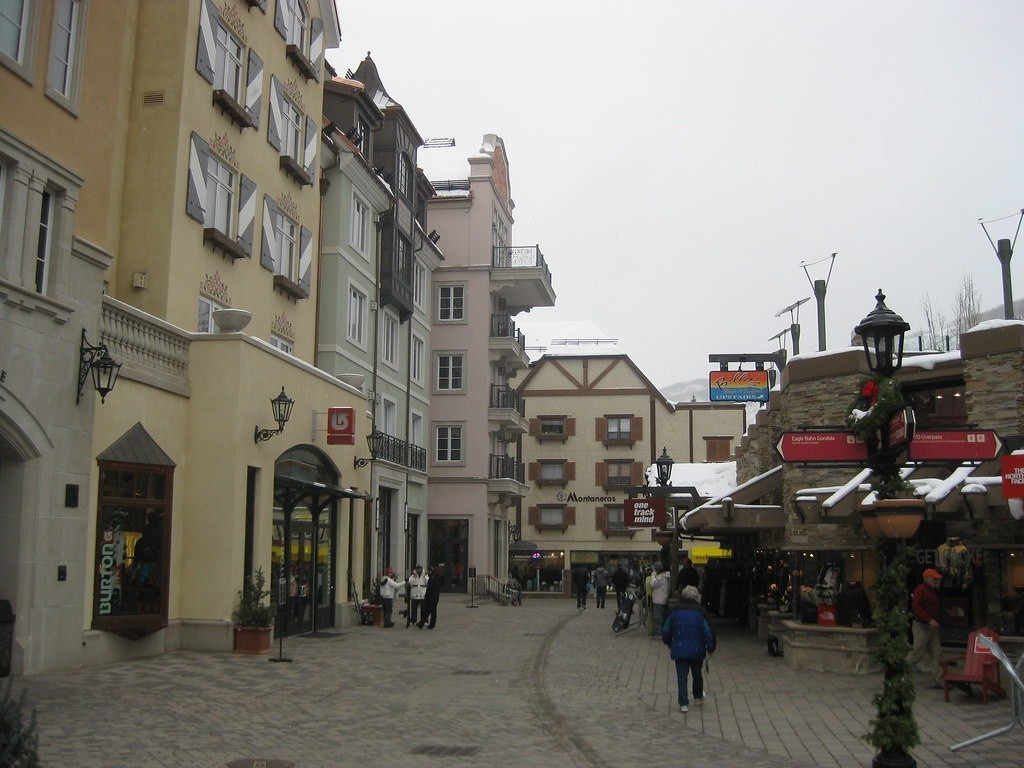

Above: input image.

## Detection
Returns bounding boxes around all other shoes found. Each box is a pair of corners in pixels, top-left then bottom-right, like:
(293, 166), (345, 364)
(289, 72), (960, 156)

(424, 622), (429, 626)
(427, 625), (435, 630)
(415, 623), (422, 629)
(409, 621), (416, 626)
(680, 692), (706, 712)
(577, 604), (587, 609)
(384, 622), (396, 628)
(932, 681), (953, 689)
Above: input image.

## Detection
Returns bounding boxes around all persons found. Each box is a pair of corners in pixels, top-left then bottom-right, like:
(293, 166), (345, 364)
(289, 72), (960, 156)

(574, 563), (590, 610)
(380, 568), (406, 628)
(409, 563), (441, 630)
(612, 564), (629, 608)
(638, 561), (670, 640)
(662, 586), (717, 712)
(932, 537), (975, 591)
(507, 577), (522, 607)
(593, 562), (611, 609)
(280, 563), (311, 625)
(904, 569), (953, 689)
(677, 559), (722, 617)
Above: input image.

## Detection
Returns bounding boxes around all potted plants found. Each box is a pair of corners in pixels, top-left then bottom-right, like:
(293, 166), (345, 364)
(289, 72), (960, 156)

(232, 564), (279, 654)
(868, 474), (924, 538)
(362, 573), (400, 626)
(656, 528), (674, 545)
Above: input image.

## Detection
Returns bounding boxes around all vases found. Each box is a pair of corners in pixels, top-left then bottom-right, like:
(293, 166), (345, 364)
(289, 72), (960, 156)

(273, 275), (305, 299)
(212, 308), (252, 332)
(336, 373), (365, 389)
(286, 44), (316, 79)
(213, 89), (252, 128)
(856, 504), (885, 542)
(204, 228), (245, 259)
(280, 155), (310, 185)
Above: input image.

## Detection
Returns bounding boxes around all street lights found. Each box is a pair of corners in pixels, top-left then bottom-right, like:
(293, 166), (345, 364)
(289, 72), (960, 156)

(854, 289), (933, 768)
(655, 446), (675, 571)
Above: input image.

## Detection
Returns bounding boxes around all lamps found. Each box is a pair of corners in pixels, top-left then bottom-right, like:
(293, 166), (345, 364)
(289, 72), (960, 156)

(849, 551), (856, 558)
(507, 523), (520, 544)
(254, 386), (295, 444)
(353, 425), (384, 470)
(75, 327), (124, 406)
(655, 446), (676, 485)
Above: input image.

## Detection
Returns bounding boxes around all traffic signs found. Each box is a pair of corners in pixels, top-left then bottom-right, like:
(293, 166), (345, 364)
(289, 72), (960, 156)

(772, 423), (1004, 467)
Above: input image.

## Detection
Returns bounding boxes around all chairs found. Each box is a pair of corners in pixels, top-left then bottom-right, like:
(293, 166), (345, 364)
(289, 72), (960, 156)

(943, 627), (1006, 705)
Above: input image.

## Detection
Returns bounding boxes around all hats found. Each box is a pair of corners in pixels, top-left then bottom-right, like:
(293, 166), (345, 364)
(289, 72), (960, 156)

(923, 569), (943, 580)
(385, 569), (397, 575)
(428, 566), (436, 571)
(415, 564), (423, 568)
